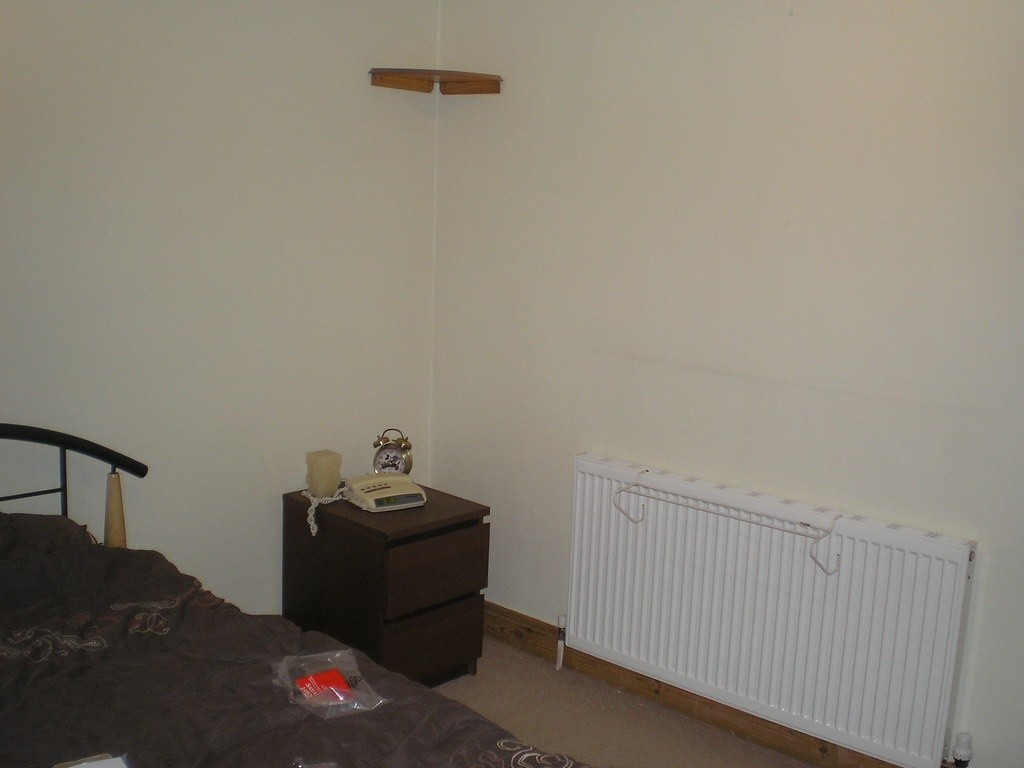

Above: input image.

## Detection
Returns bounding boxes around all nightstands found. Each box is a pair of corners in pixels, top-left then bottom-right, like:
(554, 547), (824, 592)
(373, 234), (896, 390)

(281, 474), (490, 688)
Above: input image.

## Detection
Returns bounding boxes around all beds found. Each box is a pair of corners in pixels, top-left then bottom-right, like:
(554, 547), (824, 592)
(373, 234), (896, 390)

(1, 423), (589, 768)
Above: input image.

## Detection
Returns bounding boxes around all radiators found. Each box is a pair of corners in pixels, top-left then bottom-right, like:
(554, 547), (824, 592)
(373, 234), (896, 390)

(564, 452), (977, 767)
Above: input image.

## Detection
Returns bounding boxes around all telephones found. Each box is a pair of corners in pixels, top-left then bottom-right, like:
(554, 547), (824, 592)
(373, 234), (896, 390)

(341, 472), (428, 512)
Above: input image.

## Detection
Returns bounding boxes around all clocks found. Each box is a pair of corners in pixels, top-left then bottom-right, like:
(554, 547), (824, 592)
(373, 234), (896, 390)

(372, 427), (413, 475)
